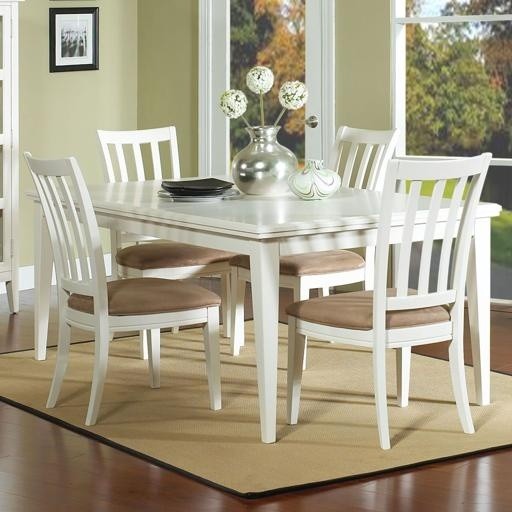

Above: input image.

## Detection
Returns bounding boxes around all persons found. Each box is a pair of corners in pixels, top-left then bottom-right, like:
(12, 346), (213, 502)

(61, 30), (86, 56)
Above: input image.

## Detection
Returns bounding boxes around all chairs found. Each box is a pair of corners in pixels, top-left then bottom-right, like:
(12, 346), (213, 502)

(95, 127), (243, 360)
(23, 149), (222, 427)
(230, 126), (402, 369)
(287, 152), (492, 451)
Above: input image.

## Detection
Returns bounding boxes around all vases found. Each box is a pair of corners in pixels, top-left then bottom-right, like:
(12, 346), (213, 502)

(231, 124), (297, 196)
(289, 157), (343, 199)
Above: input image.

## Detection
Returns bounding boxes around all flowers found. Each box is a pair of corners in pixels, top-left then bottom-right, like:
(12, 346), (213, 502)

(217, 64), (309, 128)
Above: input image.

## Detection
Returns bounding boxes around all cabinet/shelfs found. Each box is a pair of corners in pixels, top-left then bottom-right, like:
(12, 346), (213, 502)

(0, 0), (25, 313)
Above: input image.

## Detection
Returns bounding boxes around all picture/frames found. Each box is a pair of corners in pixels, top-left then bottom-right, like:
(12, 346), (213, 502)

(49, 7), (99, 72)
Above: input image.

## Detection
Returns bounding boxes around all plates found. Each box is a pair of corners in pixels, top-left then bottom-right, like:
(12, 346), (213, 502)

(157, 177), (239, 203)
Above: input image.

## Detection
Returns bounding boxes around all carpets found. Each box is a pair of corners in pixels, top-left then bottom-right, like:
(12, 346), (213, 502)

(0, 316), (512, 500)
